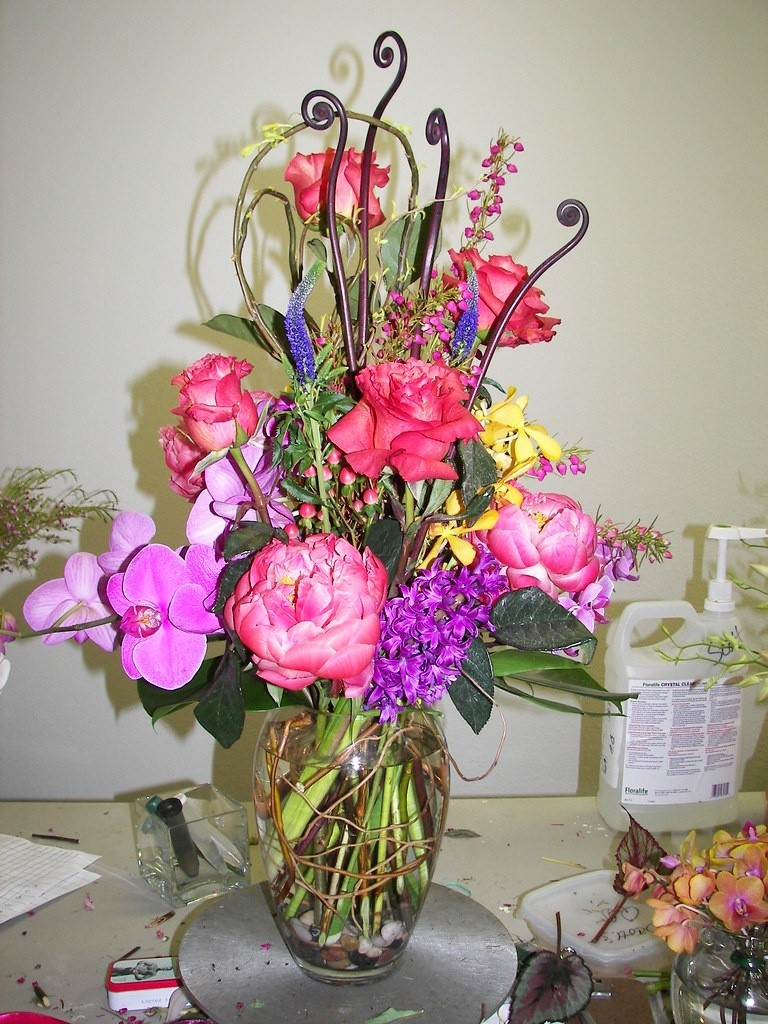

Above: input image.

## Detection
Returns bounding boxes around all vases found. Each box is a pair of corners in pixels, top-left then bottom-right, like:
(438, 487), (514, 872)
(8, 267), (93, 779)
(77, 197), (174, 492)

(666, 928), (767, 1024)
(251, 706), (454, 988)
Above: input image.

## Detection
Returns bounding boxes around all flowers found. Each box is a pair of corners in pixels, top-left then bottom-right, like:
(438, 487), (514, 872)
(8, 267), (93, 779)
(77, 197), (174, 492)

(1, 27), (676, 968)
(618, 819), (768, 1023)
(651, 528), (768, 703)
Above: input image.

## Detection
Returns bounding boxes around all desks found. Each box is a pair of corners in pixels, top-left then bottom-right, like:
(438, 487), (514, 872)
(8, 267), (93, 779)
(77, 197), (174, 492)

(2, 789), (768, 1022)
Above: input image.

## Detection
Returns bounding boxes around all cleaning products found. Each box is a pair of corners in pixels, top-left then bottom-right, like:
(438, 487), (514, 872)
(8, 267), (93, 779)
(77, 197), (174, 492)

(597, 523), (767, 830)
